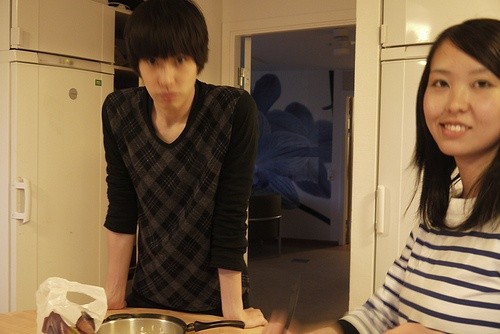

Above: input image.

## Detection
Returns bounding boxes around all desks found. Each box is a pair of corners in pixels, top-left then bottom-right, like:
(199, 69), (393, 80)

(0, 307), (266, 334)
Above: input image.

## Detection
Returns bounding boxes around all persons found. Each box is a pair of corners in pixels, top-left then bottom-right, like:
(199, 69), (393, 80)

(100, 0), (269, 328)
(291, 18), (500, 334)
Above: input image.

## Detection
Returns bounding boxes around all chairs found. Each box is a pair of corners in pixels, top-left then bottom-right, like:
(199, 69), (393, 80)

(248, 193), (282, 256)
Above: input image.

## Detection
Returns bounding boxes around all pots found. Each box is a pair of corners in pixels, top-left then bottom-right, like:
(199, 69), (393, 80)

(96, 313), (245, 334)
